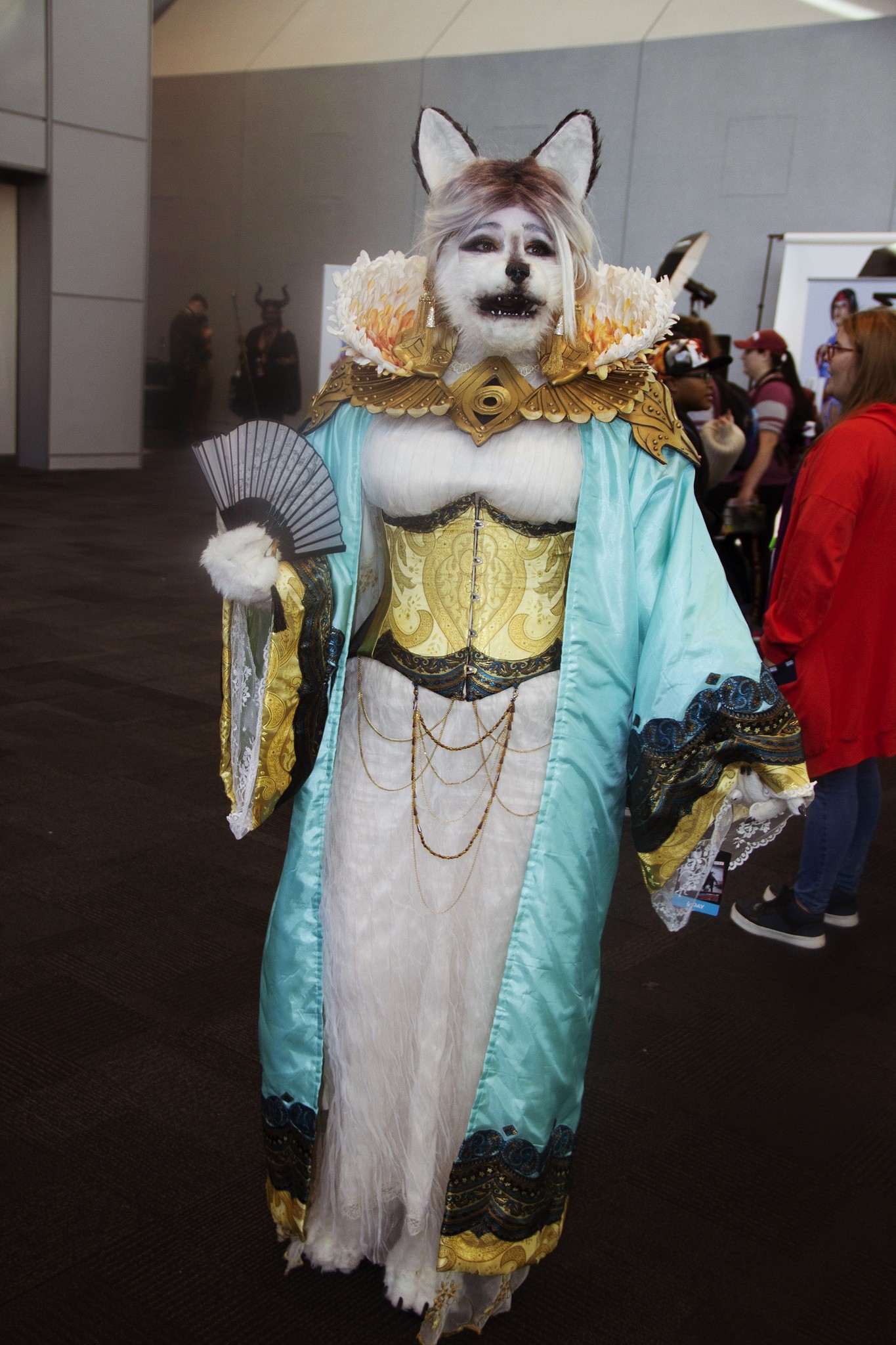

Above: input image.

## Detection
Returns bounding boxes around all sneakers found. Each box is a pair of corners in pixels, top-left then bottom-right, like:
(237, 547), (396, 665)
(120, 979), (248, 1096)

(729, 887), (826, 949)
(762, 883), (858, 928)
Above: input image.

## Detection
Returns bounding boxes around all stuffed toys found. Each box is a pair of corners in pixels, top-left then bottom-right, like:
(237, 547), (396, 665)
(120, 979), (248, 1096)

(197, 105), (813, 1345)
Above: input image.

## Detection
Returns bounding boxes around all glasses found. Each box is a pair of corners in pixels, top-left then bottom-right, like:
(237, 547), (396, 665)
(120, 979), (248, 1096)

(671, 371), (711, 381)
(828, 346), (864, 359)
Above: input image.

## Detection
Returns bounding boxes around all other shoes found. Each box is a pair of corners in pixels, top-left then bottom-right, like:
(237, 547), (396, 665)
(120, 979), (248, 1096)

(751, 631), (762, 641)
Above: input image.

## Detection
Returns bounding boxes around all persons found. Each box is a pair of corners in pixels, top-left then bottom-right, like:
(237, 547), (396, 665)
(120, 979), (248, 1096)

(815, 289), (859, 430)
(648, 318), (820, 641)
(731, 312), (896, 949)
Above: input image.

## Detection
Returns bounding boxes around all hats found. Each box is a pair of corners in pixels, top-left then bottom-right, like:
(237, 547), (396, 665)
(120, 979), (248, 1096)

(654, 338), (711, 374)
(733, 329), (786, 355)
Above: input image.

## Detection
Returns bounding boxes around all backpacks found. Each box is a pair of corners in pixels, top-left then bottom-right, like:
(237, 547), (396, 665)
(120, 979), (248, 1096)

(759, 374), (814, 464)
